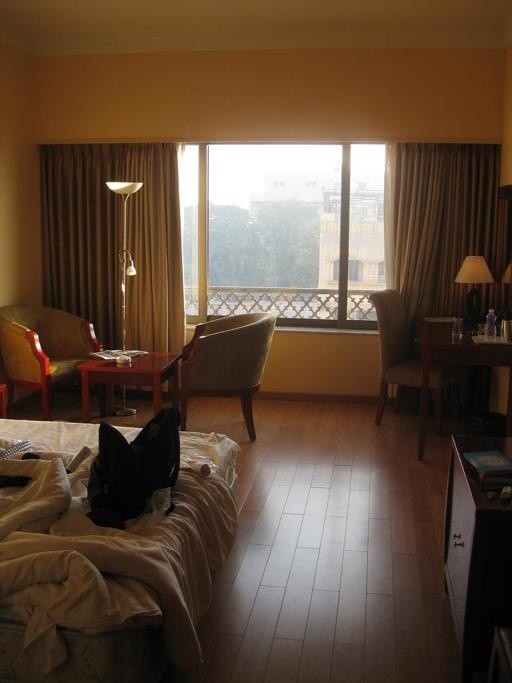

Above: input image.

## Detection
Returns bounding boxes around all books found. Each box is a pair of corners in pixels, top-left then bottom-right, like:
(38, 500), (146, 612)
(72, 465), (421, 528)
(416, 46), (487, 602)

(461, 448), (512, 493)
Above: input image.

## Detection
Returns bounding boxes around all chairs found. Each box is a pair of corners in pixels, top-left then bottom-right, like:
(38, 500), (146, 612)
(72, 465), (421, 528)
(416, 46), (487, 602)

(178, 311), (277, 441)
(369, 287), (456, 463)
(0, 307), (103, 421)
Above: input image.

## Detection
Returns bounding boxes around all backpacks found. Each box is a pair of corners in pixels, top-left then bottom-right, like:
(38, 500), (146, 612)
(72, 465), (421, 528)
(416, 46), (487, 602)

(87, 406), (180, 529)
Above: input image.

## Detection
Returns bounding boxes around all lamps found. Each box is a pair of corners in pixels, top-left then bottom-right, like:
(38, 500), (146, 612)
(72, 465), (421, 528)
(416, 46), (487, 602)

(107, 180), (146, 418)
(454, 256), (498, 322)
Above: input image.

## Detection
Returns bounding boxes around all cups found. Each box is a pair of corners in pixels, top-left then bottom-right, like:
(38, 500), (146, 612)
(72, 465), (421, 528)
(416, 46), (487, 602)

(451, 317), (464, 338)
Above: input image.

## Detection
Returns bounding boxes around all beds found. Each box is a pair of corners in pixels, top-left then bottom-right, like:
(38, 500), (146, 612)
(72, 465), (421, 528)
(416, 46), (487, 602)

(1, 419), (238, 682)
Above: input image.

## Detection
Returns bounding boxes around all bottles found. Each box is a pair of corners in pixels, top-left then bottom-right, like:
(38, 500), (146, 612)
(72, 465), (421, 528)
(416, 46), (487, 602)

(484, 308), (498, 340)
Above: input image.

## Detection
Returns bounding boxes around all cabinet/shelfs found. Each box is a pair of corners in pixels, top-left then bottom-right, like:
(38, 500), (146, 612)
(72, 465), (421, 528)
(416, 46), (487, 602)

(441, 433), (512, 683)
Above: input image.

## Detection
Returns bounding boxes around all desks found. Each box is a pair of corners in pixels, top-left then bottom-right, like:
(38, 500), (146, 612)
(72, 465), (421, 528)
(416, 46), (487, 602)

(413, 334), (511, 463)
(75, 351), (188, 432)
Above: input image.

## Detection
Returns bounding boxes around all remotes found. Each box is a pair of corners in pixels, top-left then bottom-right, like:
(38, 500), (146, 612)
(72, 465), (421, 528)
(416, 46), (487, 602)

(65, 446), (91, 473)
